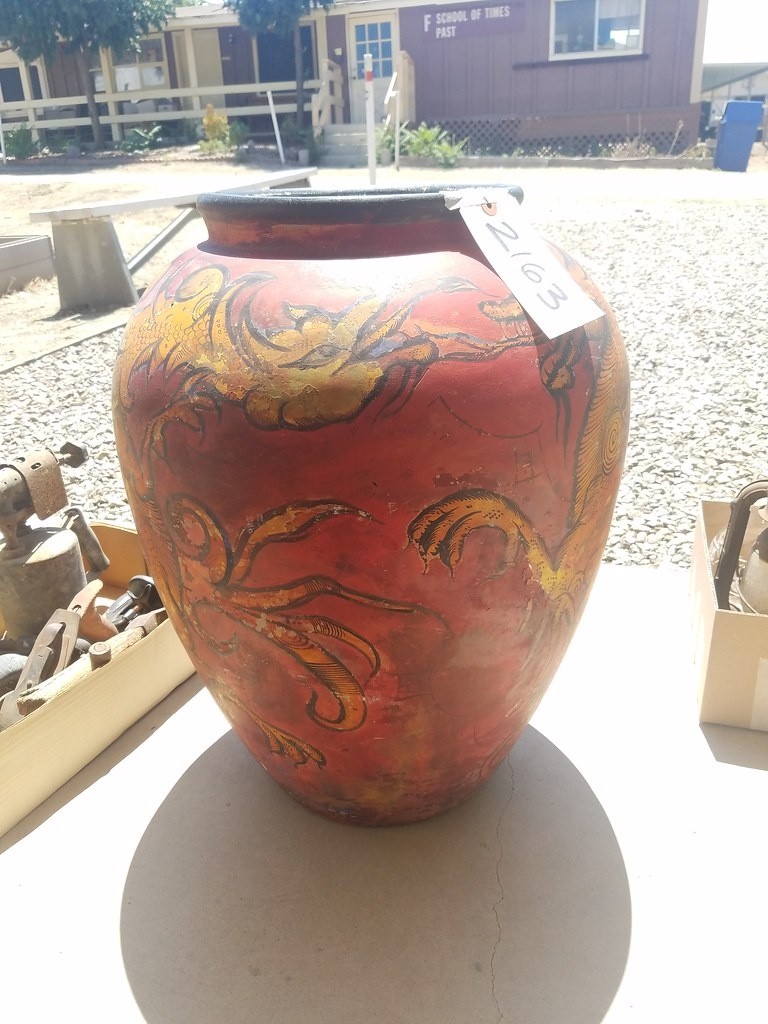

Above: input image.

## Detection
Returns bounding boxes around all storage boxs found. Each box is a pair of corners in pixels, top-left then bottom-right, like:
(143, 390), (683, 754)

(0, 520), (198, 838)
(693, 499), (768, 730)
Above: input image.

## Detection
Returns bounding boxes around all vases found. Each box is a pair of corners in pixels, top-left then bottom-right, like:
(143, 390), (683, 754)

(114, 186), (631, 833)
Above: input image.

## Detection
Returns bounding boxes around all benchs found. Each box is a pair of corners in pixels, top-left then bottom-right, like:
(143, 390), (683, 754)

(29, 165), (320, 309)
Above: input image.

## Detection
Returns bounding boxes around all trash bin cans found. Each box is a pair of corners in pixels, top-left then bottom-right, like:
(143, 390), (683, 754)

(714, 100), (764, 172)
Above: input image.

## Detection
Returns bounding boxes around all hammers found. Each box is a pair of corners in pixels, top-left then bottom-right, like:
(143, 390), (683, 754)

(15, 607), (169, 717)
